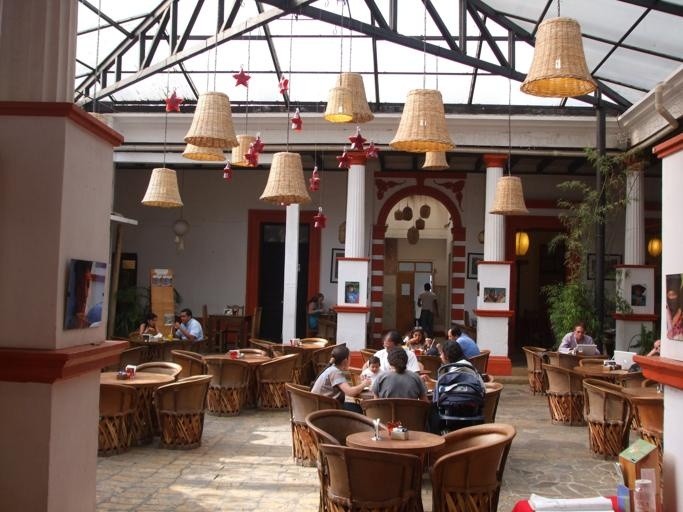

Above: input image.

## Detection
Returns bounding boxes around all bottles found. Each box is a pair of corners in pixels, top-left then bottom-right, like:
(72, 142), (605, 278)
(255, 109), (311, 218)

(633, 479), (656, 512)
(396, 425), (403, 432)
(118, 371), (123, 376)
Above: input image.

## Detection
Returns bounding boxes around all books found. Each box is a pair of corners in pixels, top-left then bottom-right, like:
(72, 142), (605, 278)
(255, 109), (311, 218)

(527, 492), (616, 512)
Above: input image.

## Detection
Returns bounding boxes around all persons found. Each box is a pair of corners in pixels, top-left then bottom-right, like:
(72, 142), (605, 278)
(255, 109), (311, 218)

(67, 261), (92, 329)
(346, 285), (356, 303)
(557, 319), (601, 356)
(666, 287), (683, 341)
(139, 313), (159, 361)
(632, 288), (646, 306)
(646, 339), (660, 356)
(497, 291), (505, 303)
(417, 283), (439, 336)
(485, 289), (497, 302)
(309, 325), (487, 415)
(173, 308), (204, 342)
(308, 293), (325, 330)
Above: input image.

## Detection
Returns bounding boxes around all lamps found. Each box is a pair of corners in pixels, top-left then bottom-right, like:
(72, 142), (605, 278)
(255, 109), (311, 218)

(647, 233), (660, 264)
(394, 195), (430, 244)
(515, 230), (529, 351)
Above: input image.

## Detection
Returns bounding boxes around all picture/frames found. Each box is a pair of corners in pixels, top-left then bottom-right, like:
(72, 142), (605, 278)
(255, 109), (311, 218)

(482, 287), (506, 304)
(343, 281), (359, 304)
(329, 247), (345, 283)
(585, 252), (623, 282)
(466, 252), (484, 280)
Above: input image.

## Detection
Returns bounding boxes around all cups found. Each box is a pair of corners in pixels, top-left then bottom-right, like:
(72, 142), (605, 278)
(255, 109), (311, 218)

(168, 334), (173, 341)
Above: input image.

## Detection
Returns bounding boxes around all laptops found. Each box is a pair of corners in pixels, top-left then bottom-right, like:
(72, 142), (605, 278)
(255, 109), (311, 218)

(614, 350), (637, 370)
(577, 343), (598, 357)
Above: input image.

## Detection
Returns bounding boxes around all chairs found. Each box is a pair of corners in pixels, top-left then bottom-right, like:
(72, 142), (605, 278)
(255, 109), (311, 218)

(305, 303), (336, 344)
(201, 303), (262, 352)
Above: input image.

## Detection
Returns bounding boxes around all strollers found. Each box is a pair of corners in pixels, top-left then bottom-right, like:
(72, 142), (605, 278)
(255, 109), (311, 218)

(429, 363), (487, 434)
